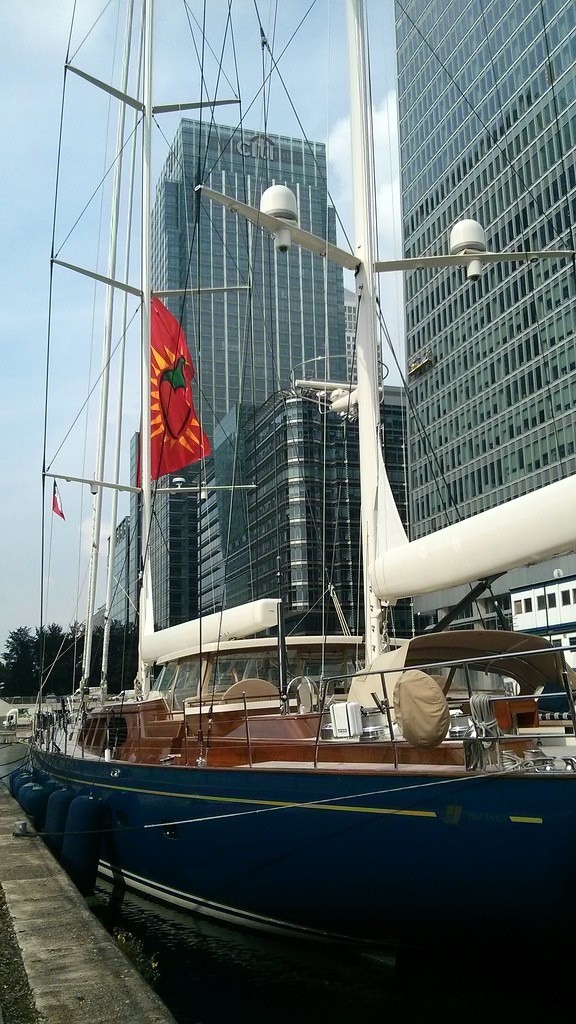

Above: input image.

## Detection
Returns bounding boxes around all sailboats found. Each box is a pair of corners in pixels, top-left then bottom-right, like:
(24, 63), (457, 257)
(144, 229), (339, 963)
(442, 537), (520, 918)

(9, 0), (576, 977)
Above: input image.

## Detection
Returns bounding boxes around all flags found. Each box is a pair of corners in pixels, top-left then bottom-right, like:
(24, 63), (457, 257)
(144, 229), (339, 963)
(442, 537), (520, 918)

(52, 482), (65, 520)
(137, 297), (211, 485)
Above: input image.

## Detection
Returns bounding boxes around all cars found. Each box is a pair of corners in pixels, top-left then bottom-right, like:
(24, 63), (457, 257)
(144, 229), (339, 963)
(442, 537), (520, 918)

(45, 695), (57, 704)
(107, 693), (117, 701)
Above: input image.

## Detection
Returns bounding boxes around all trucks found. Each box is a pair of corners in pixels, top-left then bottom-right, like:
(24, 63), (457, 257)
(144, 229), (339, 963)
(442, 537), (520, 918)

(69, 686), (105, 701)
(113, 690), (137, 703)
(3, 708), (33, 731)
